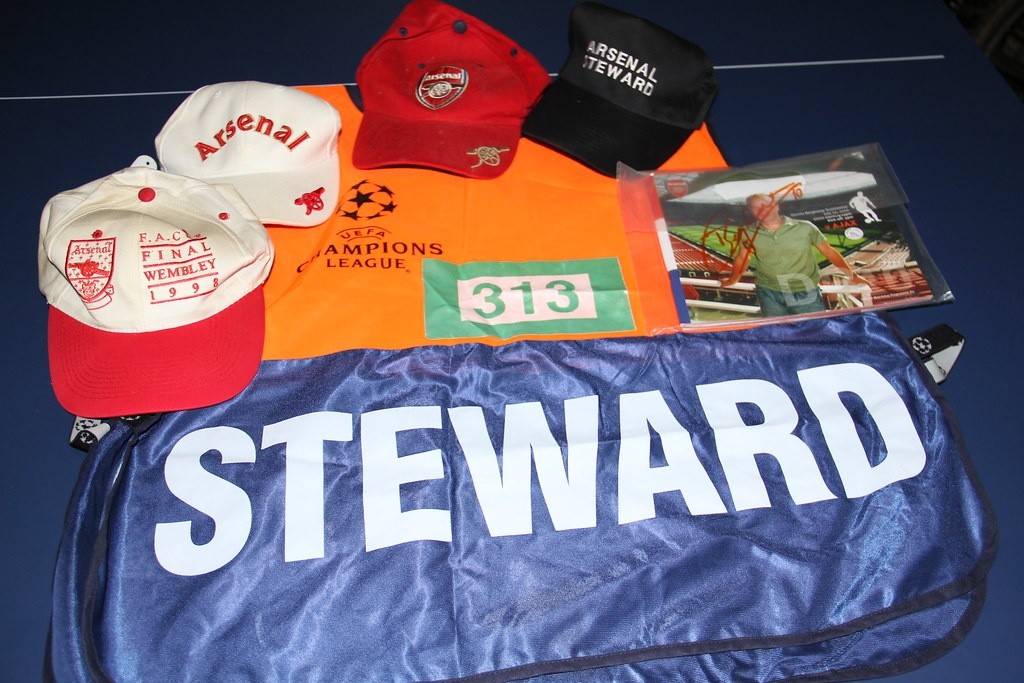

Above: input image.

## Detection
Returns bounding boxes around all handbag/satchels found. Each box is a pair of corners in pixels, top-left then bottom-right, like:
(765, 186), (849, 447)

(41, 309), (999, 683)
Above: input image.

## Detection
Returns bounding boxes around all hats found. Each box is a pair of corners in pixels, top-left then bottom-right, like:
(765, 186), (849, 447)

(38, 154), (276, 419)
(351, 0), (553, 180)
(521, 1), (720, 180)
(154, 80), (342, 227)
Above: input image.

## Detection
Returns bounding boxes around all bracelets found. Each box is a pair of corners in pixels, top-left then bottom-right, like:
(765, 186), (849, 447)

(853, 270), (859, 276)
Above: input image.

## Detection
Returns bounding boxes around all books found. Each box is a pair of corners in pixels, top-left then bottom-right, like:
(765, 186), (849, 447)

(647, 144), (933, 328)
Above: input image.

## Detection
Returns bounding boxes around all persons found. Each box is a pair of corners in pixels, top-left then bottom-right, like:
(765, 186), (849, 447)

(719, 194), (872, 319)
